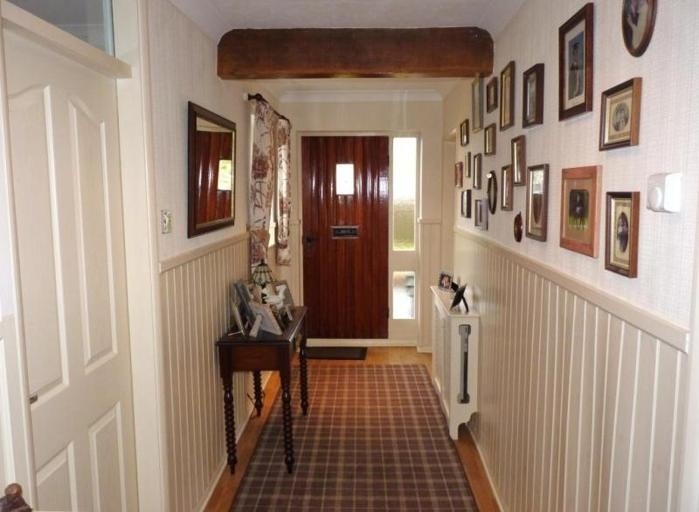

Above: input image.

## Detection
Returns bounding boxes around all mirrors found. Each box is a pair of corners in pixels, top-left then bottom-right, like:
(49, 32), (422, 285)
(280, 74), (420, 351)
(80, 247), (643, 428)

(187, 101), (236, 239)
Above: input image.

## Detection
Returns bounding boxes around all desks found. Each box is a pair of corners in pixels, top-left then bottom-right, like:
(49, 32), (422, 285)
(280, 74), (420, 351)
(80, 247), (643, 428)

(215, 306), (309, 474)
(430, 285), (480, 440)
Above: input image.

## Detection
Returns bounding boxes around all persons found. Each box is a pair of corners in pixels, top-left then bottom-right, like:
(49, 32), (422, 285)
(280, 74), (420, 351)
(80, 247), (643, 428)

(618, 216), (628, 251)
(568, 41), (581, 98)
(574, 192), (585, 232)
(615, 108), (628, 128)
(625, 0), (640, 46)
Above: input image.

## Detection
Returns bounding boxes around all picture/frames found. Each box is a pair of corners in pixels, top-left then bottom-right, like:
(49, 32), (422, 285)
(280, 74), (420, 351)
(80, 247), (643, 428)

(604, 190), (640, 278)
(621, 0), (657, 59)
(248, 302), (283, 336)
(438, 272), (454, 290)
(234, 278), (252, 313)
(455, 61), (549, 242)
(560, 165), (602, 260)
(558, 2), (594, 121)
(598, 76), (643, 151)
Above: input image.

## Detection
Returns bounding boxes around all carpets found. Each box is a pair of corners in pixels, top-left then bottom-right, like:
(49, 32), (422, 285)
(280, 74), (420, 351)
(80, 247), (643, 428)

(229, 364), (479, 512)
(298, 347), (367, 360)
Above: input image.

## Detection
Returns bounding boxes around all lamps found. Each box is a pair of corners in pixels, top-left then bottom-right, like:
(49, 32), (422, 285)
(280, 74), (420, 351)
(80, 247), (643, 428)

(251, 258), (278, 302)
